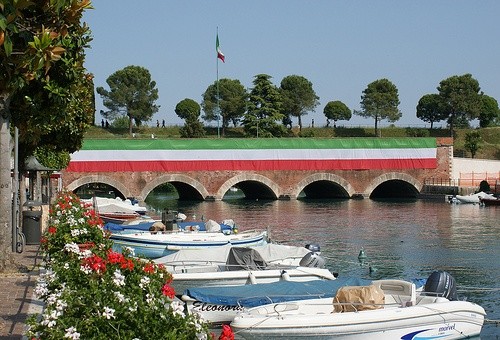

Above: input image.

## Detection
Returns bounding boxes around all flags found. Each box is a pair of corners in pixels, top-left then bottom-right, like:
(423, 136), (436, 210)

(216, 34), (225, 62)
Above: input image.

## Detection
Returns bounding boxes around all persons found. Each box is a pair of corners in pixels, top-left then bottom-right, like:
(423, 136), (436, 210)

(312, 119), (314, 127)
(25, 187), (29, 195)
(162, 120), (165, 127)
(149, 222), (166, 234)
(333, 120), (337, 128)
(102, 119), (109, 127)
(156, 119), (160, 128)
(325, 119), (330, 128)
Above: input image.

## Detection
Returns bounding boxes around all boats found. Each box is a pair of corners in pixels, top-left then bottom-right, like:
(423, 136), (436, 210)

(79, 196), (487, 340)
(455, 191), (500, 205)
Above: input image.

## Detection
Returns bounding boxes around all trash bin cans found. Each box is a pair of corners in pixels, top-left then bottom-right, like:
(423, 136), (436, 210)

(22, 200), (43, 245)
